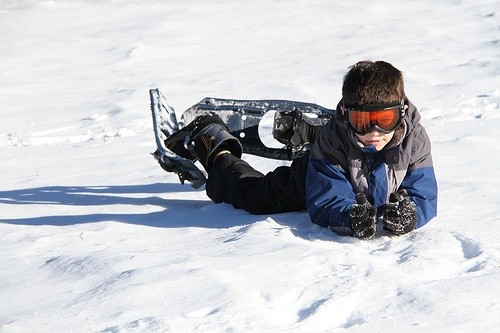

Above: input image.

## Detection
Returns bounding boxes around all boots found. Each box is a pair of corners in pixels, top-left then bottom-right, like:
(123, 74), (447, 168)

(156, 112), (243, 173)
(276, 107), (329, 152)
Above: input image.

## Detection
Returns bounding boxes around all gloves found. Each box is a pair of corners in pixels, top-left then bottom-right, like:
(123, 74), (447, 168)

(383, 192), (417, 235)
(348, 193), (377, 240)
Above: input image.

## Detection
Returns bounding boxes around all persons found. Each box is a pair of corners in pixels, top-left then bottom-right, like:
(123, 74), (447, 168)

(156, 61), (438, 241)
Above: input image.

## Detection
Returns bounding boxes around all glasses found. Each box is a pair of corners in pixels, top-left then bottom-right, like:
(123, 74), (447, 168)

(340, 99), (410, 136)
